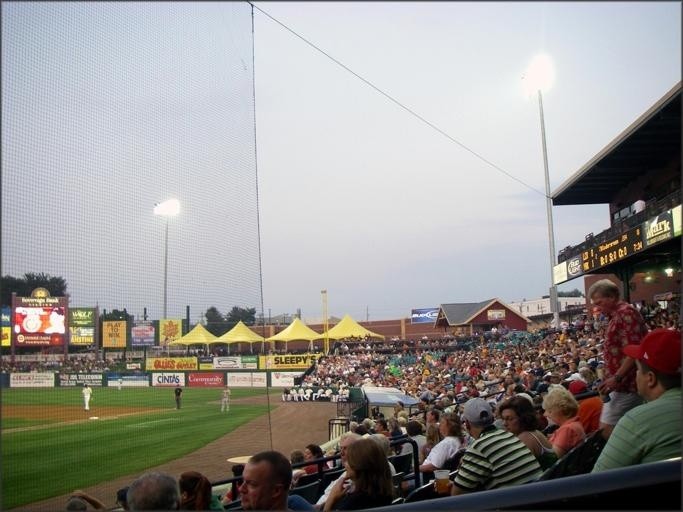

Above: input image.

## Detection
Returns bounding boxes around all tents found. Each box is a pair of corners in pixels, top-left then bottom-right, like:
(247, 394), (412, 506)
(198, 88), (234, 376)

(168, 322), (218, 357)
(264, 317), (321, 355)
(212, 319), (265, 356)
(316, 315), (385, 355)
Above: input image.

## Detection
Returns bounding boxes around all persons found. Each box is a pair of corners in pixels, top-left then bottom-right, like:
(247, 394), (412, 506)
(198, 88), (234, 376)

(2, 352), (120, 373)
(221, 385), (231, 412)
(499, 395), (559, 472)
(238, 450), (316, 510)
(126, 471), (180, 510)
(289, 443), (342, 490)
(156, 344), (322, 358)
(588, 279), (649, 440)
(590, 328), (681, 474)
(282, 297), (682, 410)
(82, 384), (93, 411)
(568, 381), (603, 435)
(178, 471), (225, 510)
(541, 387), (586, 459)
(434, 397), (560, 497)
(66, 489), (106, 510)
(316, 398), (445, 511)
(115, 486), (130, 510)
(126, 353), (133, 362)
(217, 464), (246, 505)
(117, 376), (123, 390)
(174, 384), (183, 410)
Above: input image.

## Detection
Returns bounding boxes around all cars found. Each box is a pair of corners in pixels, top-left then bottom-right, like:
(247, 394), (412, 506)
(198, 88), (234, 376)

(433, 470), (450, 493)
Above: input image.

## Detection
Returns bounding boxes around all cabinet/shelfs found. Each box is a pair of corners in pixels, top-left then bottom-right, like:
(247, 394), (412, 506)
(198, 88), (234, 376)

(594, 377), (611, 403)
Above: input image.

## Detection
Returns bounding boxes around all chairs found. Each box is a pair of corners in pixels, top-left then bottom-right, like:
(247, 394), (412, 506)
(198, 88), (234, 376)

(220, 310), (682, 512)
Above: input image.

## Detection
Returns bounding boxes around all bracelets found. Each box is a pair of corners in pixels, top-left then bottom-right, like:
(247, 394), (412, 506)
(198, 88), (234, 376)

(614, 374), (623, 383)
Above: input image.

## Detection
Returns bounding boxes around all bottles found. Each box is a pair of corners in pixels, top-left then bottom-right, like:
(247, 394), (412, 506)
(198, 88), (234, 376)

(459, 397), (494, 423)
(621, 328), (683, 376)
(568, 381), (588, 393)
